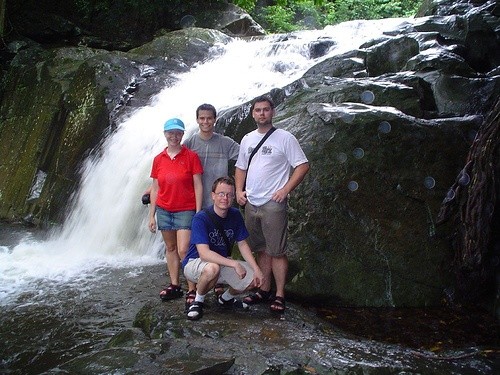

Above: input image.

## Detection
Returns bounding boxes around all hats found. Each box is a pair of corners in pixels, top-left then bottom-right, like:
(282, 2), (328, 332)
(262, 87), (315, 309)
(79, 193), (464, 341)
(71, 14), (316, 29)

(164, 118), (185, 131)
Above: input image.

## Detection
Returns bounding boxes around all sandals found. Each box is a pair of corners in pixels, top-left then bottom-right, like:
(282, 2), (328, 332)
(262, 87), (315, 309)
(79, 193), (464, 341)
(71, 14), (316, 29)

(270, 296), (286, 313)
(186, 302), (204, 319)
(243, 289), (272, 305)
(186, 290), (196, 304)
(218, 295), (249, 310)
(159, 282), (184, 300)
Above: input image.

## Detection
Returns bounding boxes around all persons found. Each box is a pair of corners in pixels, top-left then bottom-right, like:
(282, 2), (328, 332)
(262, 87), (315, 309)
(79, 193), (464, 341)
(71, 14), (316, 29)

(181, 178), (264, 320)
(144, 105), (240, 209)
(147, 118), (203, 307)
(234, 95), (309, 312)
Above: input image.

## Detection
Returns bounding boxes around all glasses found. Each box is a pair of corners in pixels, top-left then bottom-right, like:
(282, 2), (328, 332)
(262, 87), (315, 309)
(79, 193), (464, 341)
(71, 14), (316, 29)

(213, 192), (235, 198)
